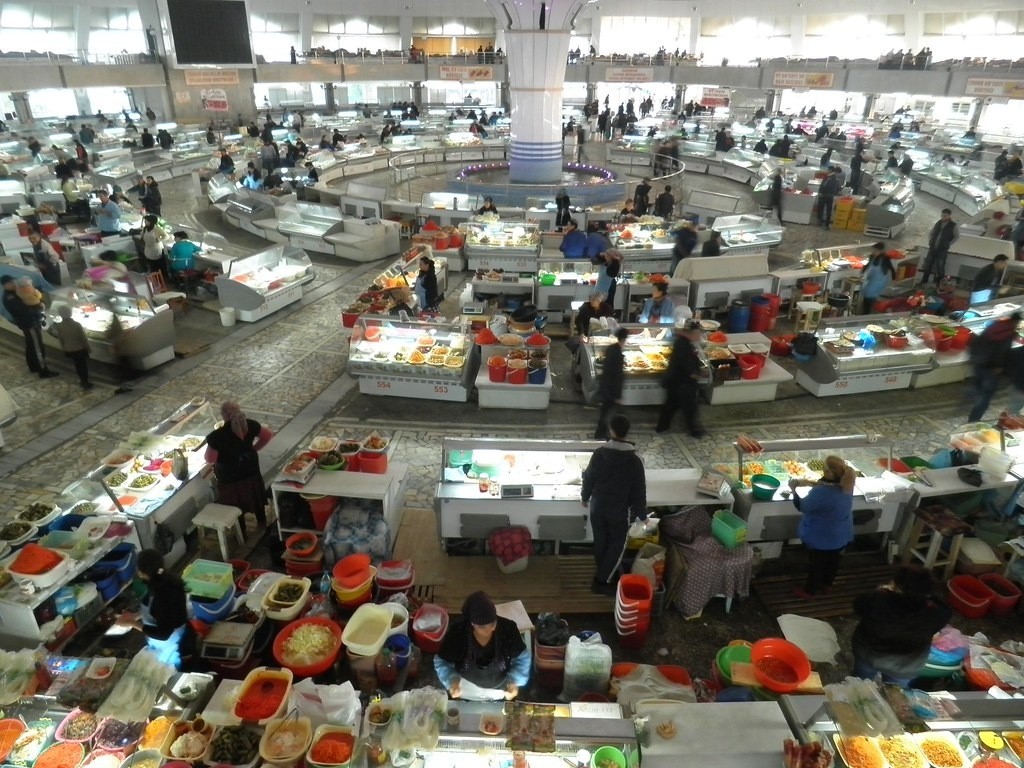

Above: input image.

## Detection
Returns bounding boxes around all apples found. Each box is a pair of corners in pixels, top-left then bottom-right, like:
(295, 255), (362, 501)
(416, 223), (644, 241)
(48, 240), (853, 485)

(785, 460), (805, 475)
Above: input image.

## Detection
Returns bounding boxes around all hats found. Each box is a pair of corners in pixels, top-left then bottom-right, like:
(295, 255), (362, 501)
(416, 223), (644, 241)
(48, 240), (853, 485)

(113, 185), (122, 192)
(0, 275), (17, 285)
(17, 275), (33, 287)
(58, 305), (73, 319)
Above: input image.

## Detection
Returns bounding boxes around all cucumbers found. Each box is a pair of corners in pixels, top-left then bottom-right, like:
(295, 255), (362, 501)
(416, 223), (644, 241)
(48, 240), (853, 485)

(210, 725), (260, 765)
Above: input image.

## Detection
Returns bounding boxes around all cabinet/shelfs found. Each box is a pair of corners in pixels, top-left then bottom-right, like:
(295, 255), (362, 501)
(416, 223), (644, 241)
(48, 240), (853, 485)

(475, 346), (552, 411)
(0, 112), (1024, 644)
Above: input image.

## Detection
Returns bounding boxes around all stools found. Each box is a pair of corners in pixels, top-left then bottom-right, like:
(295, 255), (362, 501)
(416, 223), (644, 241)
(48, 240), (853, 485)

(193, 503), (245, 563)
(794, 301), (825, 333)
(398, 218), (416, 240)
(787, 288), (814, 320)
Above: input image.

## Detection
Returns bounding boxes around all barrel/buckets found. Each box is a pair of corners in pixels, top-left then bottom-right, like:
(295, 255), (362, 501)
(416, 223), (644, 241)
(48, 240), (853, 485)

(728, 292), (782, 332)
(728, 344), (770, 378)
(219, 307), (236, 326)
(82, 567), (121, 601)
(592, 746), (626, 768)
(487, 357), (547, 384)
(922, 326), (972, 352)
(979, 574), (1021, 616)
(615, 574), (652, 646)
(947, 575), (994, 617)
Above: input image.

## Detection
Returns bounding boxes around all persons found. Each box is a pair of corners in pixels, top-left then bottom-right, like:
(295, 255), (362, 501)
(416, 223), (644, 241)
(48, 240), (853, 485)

(116, 549), (199, 673)
(0, 104), (203, 395)
(655, 323), (712, 437)
(581, 414), (649, 594)
(479, 197), (497, 215)
(356, 102), (418, 144)
(477, 42), (728, 67)
(670, 226), (721, 278)
(563, 94), (919, 230)
(448, 94), (505, 139)
(884, 47), (932, 70)
(589, 248), (623, 305)
(291, 45), (417, 65)
(639, 282), (675, 324)
(593, 328), (629, 442)
(206, 96), (347, 194)
(433, 591), (531, 702)
(555, 177), (675, 258)
(574, 291), (614, 375)
(204, 400), (273, 542)
(788, 456), (856, 595)
(860, 127), (1024, 422)
(415, 256), (438, 312)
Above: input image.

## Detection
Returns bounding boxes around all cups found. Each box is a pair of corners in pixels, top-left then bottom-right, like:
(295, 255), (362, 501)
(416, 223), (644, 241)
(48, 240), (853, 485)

(446, 707), (460, 726)
(576, 749), (591, 767)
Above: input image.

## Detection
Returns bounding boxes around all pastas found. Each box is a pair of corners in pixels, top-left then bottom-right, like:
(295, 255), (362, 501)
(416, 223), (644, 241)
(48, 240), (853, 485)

(836, 736), (963, 768)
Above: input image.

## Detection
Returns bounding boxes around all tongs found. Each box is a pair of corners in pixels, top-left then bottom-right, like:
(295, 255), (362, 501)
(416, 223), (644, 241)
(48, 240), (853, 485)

(914, 470), (932, 486)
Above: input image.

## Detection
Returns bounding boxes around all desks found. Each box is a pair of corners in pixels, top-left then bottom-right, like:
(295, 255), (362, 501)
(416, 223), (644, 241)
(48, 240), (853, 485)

(691, 540), (754, 615)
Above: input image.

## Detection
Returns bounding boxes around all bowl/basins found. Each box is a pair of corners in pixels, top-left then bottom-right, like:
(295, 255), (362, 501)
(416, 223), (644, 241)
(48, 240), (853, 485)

(802, 282), (821, 294)
(827, 293), (849, 307)
(0, 394), (409, 768)
(751, 474), (780, 500)
(926, 295), (944, 311)
(508, 304), (539, 330)
(716, 635), (812, 701)
(541, 274), (556, 285)
(479, 713), (504, 736)
(364, 702), (393, 727)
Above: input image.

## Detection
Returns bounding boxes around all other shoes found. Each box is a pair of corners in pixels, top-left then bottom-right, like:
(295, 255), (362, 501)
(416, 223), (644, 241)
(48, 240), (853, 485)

(590, 574), (609, 594)
(28, 365), (40, 373)
(39, 314), (47, 327)
(39, 365), (61, 378)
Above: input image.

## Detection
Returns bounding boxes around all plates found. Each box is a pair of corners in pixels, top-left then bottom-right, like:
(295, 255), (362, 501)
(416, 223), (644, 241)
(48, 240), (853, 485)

(472, 333), (551, 347)
(357, 336), (466, 367)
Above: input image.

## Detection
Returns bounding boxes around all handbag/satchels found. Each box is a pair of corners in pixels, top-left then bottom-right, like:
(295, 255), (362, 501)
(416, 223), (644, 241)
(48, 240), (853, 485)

(171, 448), (188, 479)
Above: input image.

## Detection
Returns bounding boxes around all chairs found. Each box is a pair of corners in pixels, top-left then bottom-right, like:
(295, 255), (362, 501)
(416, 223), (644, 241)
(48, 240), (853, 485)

(144, 269), (188, 318)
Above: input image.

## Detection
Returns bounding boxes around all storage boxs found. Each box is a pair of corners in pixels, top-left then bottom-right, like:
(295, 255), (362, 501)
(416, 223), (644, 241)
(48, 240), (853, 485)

(846, 219), (865, 231)
(851, 208), (867, 223)
(339, 601), (394, 657)
(833, 209), (851, 220)
(836, 198), (855, 210)
(832, 218), (848, 230)
(710, 509), (749, 550)
(181, 558), (233, 599)
(710, 358), (743, 382)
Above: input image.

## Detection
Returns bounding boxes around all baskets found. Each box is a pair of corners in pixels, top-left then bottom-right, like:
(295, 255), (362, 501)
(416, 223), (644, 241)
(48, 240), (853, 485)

(711, 509), (749, 550)
(888, 335), (907, 348)
